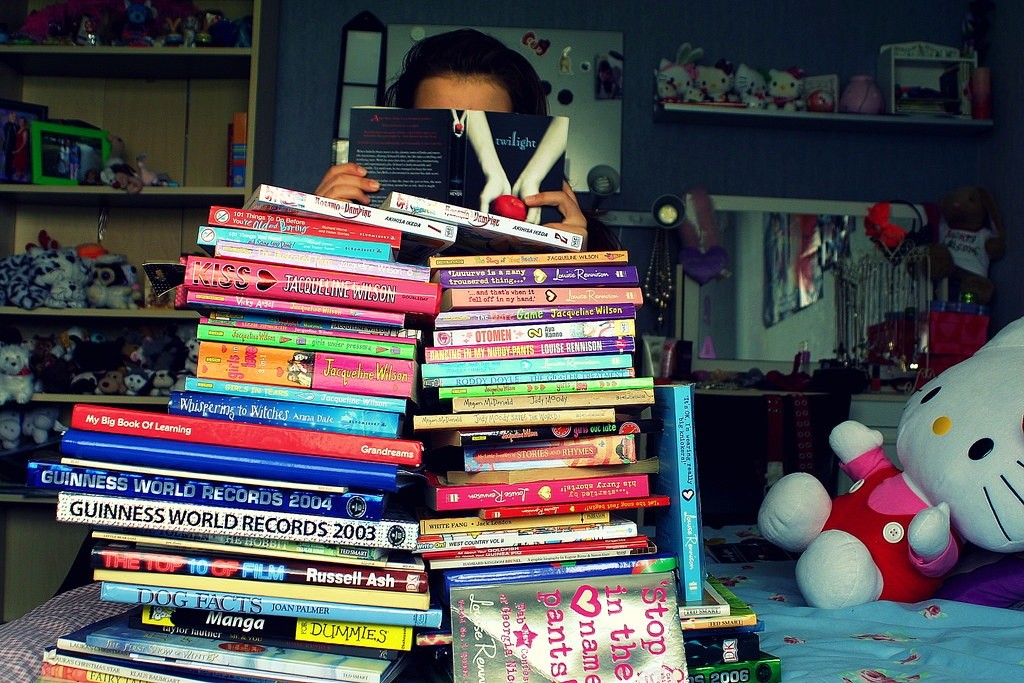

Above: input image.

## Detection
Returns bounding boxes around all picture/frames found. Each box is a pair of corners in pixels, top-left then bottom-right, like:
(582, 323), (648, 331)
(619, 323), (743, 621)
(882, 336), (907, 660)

(31, 121), (109, 184)
(0, 100), (50, 184)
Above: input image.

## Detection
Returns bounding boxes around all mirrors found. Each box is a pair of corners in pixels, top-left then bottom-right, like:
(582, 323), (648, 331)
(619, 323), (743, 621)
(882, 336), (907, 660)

(675, 196), (925, 369)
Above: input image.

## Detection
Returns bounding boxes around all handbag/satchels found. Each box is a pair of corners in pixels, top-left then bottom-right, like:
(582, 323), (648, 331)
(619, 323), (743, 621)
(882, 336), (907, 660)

(810, 367), (872, 395)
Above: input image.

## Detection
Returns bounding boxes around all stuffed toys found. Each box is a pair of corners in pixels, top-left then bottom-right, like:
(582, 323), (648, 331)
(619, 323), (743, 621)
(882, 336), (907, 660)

(11, 0), (198, 47)
(757, 314), (1023, 609)
(99, 134), (158, 194)
(656, 59), (806, 112)
(921, 184), (1006, 303)
(0, 228), (200, 449)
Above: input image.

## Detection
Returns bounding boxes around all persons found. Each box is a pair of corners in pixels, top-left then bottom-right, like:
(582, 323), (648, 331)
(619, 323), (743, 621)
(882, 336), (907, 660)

(287, 370), (312, 386)
(286, 360), (314, 377)
(451, 109), (467, 138)
(57, 138), (81, 181)
(292, 352), (315, 364)
(313, 29), (588, 252)
(596, 60), (621, 99)
(467, 110), (569, 224)
(2, 111), (29, 179)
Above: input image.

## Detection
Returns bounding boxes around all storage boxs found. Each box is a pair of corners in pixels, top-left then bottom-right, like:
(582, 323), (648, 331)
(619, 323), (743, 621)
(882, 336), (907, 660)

(916, 298), (994, 387)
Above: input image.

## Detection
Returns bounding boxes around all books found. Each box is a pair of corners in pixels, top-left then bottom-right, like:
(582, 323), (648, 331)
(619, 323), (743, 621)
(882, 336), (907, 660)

(348, 106), (570, 226)
(25, 184), (783, 683)
(229, 111), (247, 187)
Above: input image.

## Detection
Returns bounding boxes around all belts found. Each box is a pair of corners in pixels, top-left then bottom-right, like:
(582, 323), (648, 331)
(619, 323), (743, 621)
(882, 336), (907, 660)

(764, 370), (814, 487)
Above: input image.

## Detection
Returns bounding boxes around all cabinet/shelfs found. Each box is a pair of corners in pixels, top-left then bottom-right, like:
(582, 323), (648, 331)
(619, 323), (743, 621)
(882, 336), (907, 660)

(0, 0), (265, 512)
(836, 393), (916, 500)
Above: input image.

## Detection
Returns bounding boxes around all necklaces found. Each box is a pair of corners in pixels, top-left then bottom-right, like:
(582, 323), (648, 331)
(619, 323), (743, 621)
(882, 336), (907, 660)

(645, 226), (672, 320)
(834, 255), (921, 372)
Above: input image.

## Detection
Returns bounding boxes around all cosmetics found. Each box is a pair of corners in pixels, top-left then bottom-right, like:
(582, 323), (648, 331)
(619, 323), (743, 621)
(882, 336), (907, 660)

(662, 338), (678, 379)
(641, 335), (665, 377)
